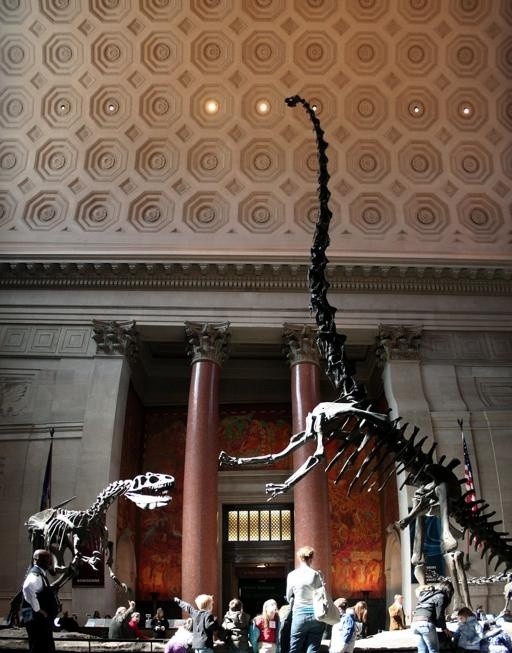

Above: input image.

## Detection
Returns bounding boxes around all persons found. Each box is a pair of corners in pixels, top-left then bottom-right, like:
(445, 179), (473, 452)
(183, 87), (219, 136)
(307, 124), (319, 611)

(451, 606), (484, 652)
(387, 594), (406, 631)
(501, 609), (512, 622)
(408, 580), (455, 652)
(127, 612), (155, 641)
(450, 606), (461, 622)
(108, 599), (139, 640)
(475, 605), (484, 621)
(21, 548), (77, 652)
(165, 593), (368, 653)
(285, 546), (327, 653)
(151, 608), (170, 639)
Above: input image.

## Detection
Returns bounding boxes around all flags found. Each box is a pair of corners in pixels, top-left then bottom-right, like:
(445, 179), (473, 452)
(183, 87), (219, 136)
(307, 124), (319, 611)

(39, 437), (54, 511)
(458, 432), (479, 514)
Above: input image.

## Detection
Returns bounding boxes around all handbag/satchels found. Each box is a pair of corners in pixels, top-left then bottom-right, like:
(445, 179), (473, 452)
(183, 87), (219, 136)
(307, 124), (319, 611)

(313, 586), (340, 625)
(21, 588), (58, 625)
